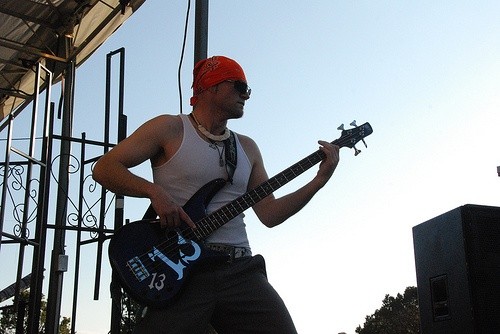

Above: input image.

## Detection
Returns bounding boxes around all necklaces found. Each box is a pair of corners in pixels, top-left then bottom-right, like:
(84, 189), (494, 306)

(207, 138), (225, 167)
(191, 113), (231, 141)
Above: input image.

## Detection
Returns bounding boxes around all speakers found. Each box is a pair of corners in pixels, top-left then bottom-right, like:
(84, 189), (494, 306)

(413, 204), (500, 334)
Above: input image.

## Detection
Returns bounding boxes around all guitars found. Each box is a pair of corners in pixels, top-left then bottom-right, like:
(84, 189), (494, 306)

(108, 119), (373, 311)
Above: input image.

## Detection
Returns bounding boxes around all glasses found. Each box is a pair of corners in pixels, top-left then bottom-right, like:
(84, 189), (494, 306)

(226, 79), (251, 95)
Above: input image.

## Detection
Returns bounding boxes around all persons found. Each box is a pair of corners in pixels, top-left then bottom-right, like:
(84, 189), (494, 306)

(91, 56), (340, 334)
